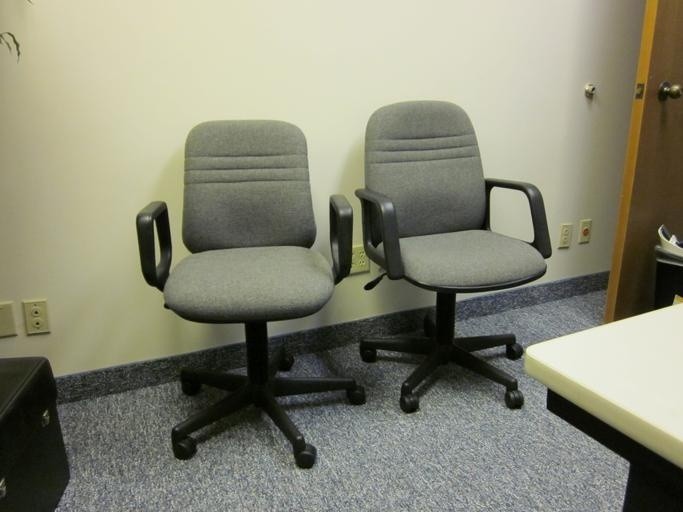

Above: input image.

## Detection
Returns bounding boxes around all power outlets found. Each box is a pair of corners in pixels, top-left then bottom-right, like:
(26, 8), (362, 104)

(349, 244), (370, 274)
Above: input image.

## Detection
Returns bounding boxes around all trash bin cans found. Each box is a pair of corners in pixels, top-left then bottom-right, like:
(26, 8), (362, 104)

(653, 225), (682, 310)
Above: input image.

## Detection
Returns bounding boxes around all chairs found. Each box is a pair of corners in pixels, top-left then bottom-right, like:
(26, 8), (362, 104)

(136, 118), (366, 467)
(354, 99), (552, 414)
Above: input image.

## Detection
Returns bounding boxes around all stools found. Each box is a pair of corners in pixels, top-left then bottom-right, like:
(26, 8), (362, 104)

(1, 357), (71, 512)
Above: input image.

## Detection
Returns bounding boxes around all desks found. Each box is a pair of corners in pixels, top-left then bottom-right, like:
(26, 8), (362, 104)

(523, 297), (683, 511)
